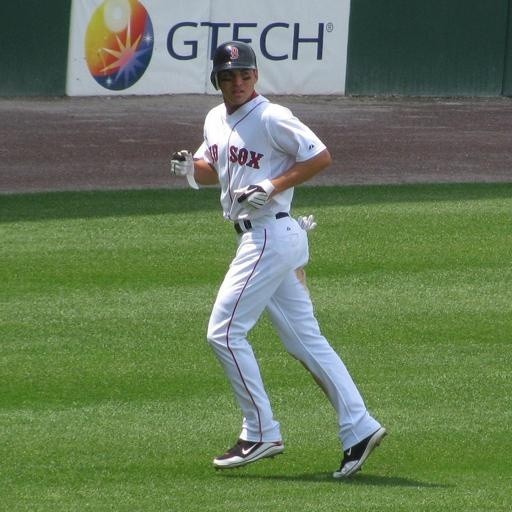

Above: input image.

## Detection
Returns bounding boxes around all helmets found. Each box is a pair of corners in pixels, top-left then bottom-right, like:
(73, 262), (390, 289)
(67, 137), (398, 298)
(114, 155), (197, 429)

(212, 42), (256, 90)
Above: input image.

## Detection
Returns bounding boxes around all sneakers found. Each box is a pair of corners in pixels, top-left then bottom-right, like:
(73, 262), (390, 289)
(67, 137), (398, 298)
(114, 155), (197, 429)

(333, 427), (386, 478)
(213, 439), (285, 469)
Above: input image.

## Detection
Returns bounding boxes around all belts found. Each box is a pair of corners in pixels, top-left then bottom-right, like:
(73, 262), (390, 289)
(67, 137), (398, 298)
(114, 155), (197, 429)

(235, 212), (289, 233)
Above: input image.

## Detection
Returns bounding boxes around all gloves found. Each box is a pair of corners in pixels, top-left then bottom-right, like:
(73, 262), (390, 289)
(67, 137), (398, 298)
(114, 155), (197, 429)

(170, 150), (193, 176)
(298, 215), (317, 230)
(234, 179), (275, 209)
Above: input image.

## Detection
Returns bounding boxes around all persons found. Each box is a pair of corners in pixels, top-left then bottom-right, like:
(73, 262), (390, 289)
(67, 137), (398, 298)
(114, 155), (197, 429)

(170, 42), (387, 480)
